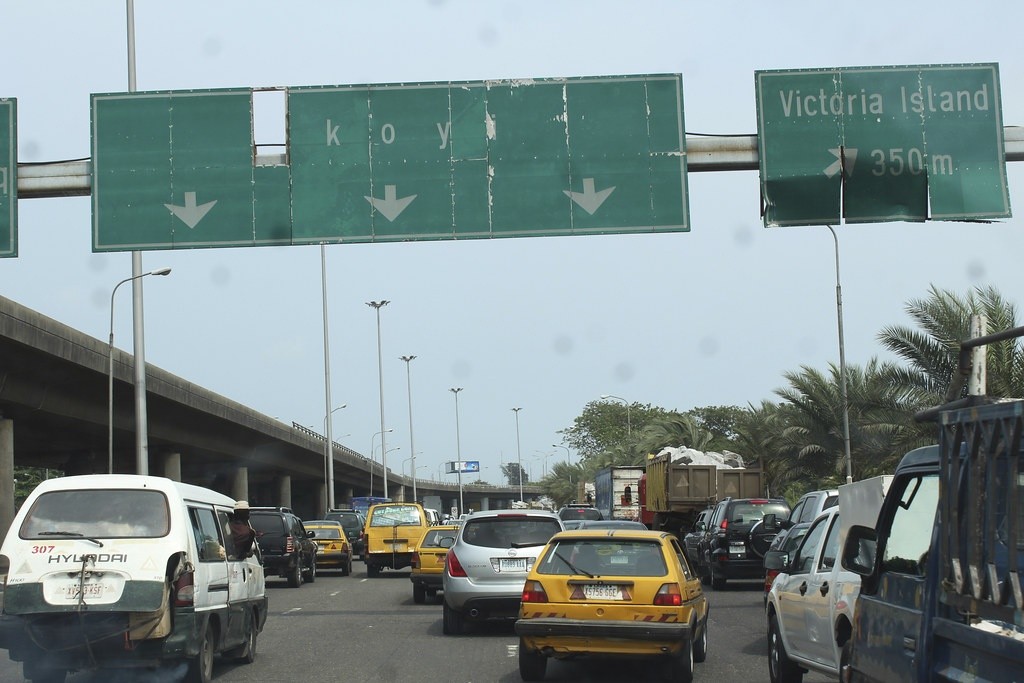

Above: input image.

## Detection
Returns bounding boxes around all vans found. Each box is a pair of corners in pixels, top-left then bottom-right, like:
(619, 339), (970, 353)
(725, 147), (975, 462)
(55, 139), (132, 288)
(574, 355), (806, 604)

(0, 472), (269, 682)
(364, 503), (438, 578)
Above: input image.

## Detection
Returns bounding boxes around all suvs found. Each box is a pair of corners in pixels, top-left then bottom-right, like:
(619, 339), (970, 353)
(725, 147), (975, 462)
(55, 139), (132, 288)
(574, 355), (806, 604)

(557, 503), (604, 530)
(323, 508), (367, 562)
(682, 489), (840, 608)
(439, 508), (572, 635)
(250, 507), (320, 588)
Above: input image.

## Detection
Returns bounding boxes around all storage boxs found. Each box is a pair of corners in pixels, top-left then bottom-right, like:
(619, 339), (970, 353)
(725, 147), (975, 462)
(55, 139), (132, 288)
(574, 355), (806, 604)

(129, 574), (170, 641)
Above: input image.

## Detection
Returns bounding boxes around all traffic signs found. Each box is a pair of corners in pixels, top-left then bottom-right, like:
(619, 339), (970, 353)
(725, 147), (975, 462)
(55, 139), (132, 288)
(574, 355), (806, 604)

(88, 75), (691, 252)
(755, 63), (1012, 226)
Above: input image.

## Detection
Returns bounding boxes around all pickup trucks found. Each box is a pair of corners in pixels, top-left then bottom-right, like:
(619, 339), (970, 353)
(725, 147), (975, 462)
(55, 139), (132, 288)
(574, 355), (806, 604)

(764, 504), (862, 683)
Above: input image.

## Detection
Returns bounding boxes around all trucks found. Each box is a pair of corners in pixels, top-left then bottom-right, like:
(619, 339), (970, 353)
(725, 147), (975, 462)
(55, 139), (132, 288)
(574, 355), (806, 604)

(587, 466), (645, 522)
(839, 392), (1024, 683)
(624, 451), (768, 541)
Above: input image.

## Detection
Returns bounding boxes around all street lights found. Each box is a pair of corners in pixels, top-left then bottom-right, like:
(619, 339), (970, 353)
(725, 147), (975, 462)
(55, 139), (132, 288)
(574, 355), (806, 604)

(449, 387), (464, 515)
(511, 407), (523, 502)
(364, 298), (391, 498)
(370, 428), (429, 504)
(323, 404), (347, 514)
(600, 394), (630, 435)
(552, 444), (572, 483)
(398, 355), (417, 503)
(107, 266), (172, 475)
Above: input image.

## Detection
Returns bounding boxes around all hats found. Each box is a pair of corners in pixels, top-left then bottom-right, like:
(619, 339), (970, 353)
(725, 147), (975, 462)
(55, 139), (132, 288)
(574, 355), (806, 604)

(233, 501), (250, 511)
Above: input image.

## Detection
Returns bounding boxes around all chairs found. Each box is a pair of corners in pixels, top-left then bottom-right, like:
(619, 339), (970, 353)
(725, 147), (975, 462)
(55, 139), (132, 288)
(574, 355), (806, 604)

(630, 555), (663, 575)
(473, 527), (503, 546)
(570, 544), (605, 573)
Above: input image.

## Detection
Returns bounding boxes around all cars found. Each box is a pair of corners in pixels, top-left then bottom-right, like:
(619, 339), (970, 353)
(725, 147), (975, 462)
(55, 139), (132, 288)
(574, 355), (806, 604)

(575, 520), (649, 531)
(301, 519), (353, 575)
(409, 524), (461, 604)
(514, 528), (714, 683)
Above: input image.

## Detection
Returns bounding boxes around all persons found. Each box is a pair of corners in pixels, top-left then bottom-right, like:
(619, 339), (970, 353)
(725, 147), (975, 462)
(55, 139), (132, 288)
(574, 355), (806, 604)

(231, 501), (255, 554)
